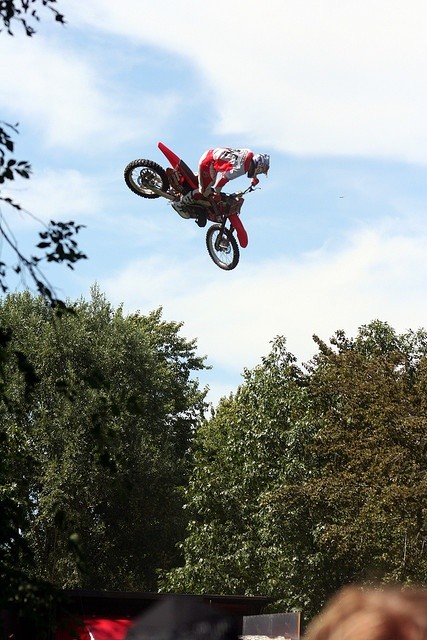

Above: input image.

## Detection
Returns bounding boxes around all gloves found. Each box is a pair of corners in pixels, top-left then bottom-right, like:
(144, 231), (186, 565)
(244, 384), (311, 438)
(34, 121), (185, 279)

(251, 178), (258, 186)
(214, 194), (222, 203)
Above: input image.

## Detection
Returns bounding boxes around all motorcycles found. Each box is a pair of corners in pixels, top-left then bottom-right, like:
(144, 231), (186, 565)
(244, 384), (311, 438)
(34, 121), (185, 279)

(124, 142), (247, 270)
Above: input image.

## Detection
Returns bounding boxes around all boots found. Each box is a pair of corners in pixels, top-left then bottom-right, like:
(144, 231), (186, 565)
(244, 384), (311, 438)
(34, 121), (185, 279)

(171, 192), (195, 219)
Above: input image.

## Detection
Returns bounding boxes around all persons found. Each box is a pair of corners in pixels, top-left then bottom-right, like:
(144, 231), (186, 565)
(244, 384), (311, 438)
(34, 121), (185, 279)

(170, 146), (271, 220)
(302, 573), (426, 639)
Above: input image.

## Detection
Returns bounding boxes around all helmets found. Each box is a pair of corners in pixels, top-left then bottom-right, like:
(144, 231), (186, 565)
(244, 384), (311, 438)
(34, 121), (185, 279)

(252, 152), (270, 174)
